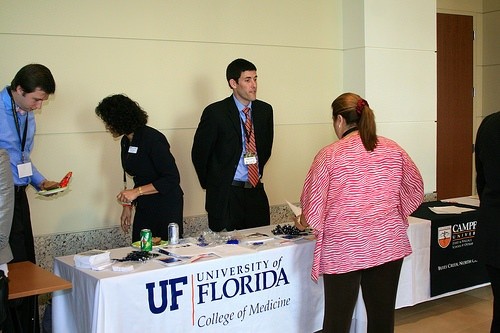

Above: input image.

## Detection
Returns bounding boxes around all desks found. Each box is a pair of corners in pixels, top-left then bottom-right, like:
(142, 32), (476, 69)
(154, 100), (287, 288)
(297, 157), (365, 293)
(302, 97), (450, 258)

(7, 260), (74, 333)
(53, 194), (491, 333)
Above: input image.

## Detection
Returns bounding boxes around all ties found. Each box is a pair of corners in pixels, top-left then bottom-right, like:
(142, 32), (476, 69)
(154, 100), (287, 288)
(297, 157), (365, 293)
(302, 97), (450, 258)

(242, 107), (259, 187)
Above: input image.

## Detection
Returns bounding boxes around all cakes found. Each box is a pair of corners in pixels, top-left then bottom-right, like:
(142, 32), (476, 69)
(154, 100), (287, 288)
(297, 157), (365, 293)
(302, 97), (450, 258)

(73, 249), (110, 269)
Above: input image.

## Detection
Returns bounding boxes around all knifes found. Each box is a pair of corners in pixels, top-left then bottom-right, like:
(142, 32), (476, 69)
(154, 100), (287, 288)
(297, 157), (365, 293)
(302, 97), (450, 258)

(157, 249), (179, 258)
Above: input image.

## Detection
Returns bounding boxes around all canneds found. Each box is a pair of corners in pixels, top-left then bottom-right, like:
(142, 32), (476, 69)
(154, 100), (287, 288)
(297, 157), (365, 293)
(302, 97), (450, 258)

(140, 229), (152, 251)
(168, 223), (179, 245)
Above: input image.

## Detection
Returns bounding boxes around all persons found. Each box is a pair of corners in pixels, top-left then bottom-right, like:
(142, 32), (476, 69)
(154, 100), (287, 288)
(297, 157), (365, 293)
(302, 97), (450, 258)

(95, 93), (184, 248)
(292, 93), (425, 333)
(0, 64), (64, 333)
(191, 58), (274, 233)
(473, 111), (500, 333)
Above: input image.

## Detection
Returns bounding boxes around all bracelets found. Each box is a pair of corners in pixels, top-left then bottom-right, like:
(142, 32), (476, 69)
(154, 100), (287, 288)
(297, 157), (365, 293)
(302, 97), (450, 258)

(297, 215), (309, 229)
(138, 186), (143, 196)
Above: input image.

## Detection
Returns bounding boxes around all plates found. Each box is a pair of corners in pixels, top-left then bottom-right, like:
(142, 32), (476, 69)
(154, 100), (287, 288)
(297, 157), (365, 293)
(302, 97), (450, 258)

(131, 239), (169, 249)
(118, 253), (160, 263)
(35, 186), (69, 197)
(274, 235), (304, 241)
(158, 243), (211, 258)
(207, 244), (256, 258)
(245, 233), (274, 244)
